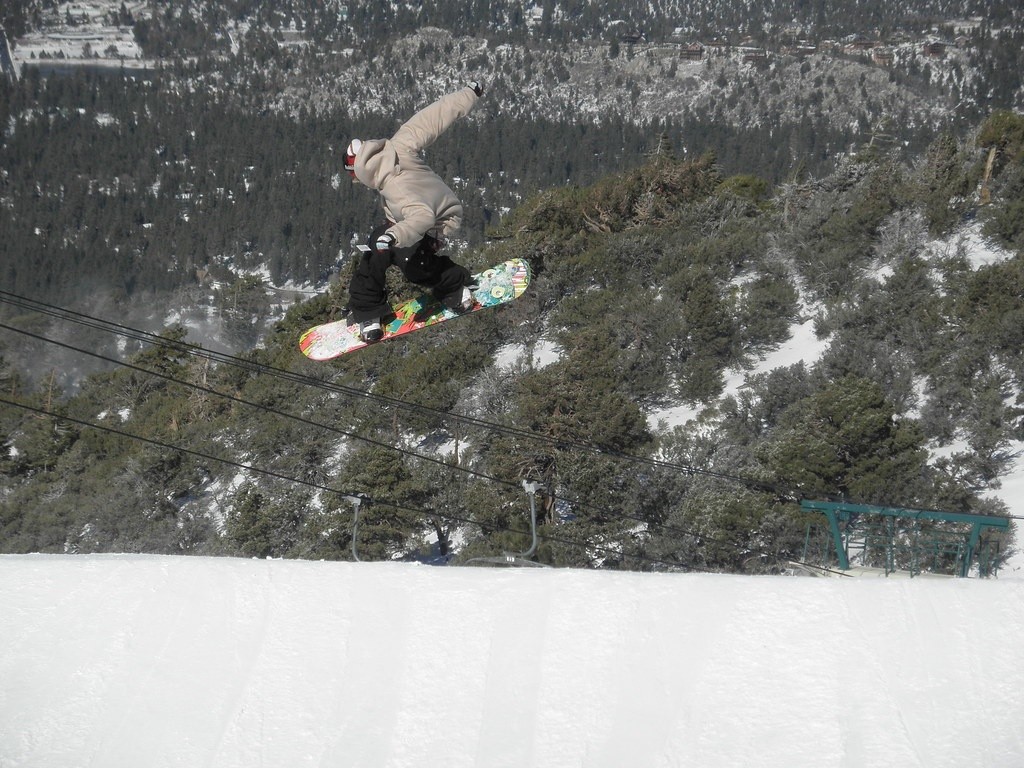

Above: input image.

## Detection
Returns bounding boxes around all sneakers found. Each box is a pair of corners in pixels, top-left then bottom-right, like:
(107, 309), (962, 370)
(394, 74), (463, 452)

(442, 286), (472, 310)
(359, 316), (382, 342)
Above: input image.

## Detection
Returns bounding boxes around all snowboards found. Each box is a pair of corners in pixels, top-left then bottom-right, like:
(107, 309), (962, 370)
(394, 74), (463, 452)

(298, 257), (532, 360)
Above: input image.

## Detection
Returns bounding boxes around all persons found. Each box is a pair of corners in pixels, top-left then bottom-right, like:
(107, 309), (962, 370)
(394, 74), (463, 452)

(341, 81), (484, 343)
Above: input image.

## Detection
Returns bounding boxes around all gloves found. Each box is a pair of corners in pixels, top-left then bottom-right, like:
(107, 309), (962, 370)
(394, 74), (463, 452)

(376, 232), (396, 254)
(468, 80), (483, 97)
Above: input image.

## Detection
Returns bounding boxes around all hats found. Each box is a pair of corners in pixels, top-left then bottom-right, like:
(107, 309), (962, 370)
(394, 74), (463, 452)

(342, 138), (367, 177)
